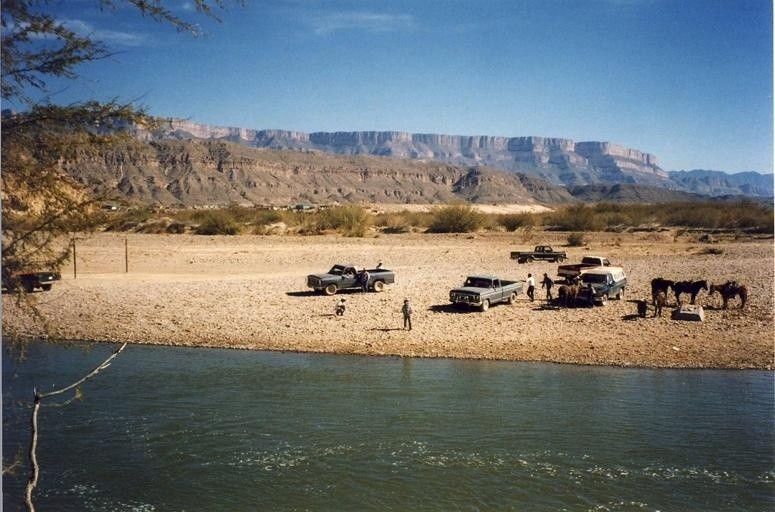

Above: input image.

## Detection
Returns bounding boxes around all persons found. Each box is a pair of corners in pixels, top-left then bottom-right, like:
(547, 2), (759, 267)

(586, 283), (597, 308)
(360, 268), (370, 293)
(571, 275), (583, 302)
(400, 300), (414, 332)
(541, 272), (555, 301)
(375, 263), (384, 270)
(333, 298), (346, 316)
(519, 272), (535, 302)
(637, 299), (650, 317)
(653, 288), (665, 317)
(346, 270), (354, 277)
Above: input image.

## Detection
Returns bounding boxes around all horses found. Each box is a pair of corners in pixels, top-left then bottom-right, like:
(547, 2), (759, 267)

(558, 279), (583, 308)
(710, 284), (747, 309)
(674, 280), (708, 305)
(651, 278), (675, 298)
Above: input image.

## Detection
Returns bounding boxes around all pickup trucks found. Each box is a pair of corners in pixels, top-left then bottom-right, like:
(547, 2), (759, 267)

(306, 264), (395, 296)
(1, 262), (60, 292)
(509, 244), (627, 307)
(450, 274), (523, 313)
